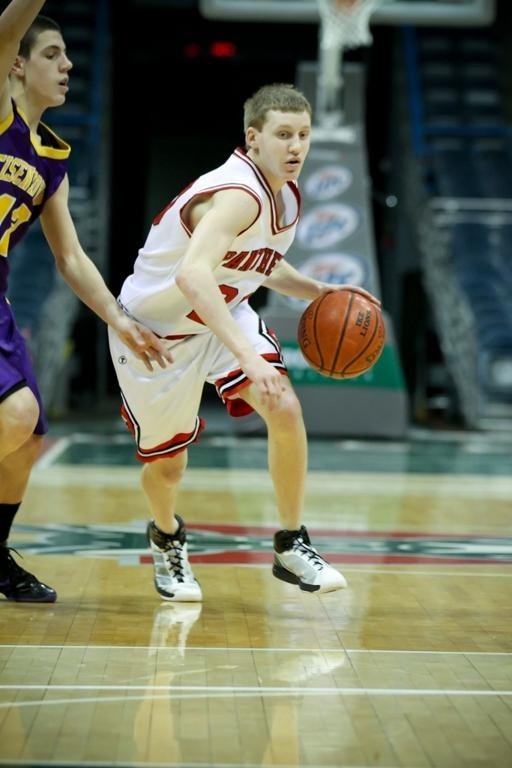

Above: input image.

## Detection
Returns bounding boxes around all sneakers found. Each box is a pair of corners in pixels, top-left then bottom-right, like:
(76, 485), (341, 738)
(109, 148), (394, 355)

(272, 524), (348, 593)
(0, 541), (56, 602)
(147, 511), (203, 602)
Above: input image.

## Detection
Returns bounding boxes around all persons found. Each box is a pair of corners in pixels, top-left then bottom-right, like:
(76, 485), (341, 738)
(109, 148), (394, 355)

(108, 82), (383, 604)
(0, 0), (175, 605)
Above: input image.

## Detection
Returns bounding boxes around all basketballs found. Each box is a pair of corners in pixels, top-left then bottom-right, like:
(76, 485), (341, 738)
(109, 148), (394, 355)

(300, 288), (384, 377)
